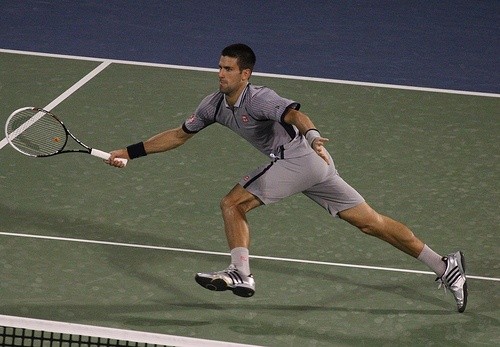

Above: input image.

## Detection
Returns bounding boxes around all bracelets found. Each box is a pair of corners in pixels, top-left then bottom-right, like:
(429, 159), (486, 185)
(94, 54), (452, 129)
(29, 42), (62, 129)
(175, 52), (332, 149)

(127, 141), (148, 160)
(304, 128), (321, 148)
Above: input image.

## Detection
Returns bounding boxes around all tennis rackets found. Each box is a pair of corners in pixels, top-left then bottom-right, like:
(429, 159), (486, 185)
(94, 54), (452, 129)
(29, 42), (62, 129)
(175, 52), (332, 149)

(4, 106), (128, 167)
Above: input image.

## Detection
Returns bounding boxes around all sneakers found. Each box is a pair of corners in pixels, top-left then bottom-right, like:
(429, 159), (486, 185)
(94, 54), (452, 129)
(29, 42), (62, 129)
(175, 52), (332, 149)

(195, 263), (255, 298)
(435, 250), (468, 313)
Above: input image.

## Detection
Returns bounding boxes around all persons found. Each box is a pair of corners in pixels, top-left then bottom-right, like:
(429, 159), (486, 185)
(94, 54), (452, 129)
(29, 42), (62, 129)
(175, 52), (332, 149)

(103, 43), (468, 312)
